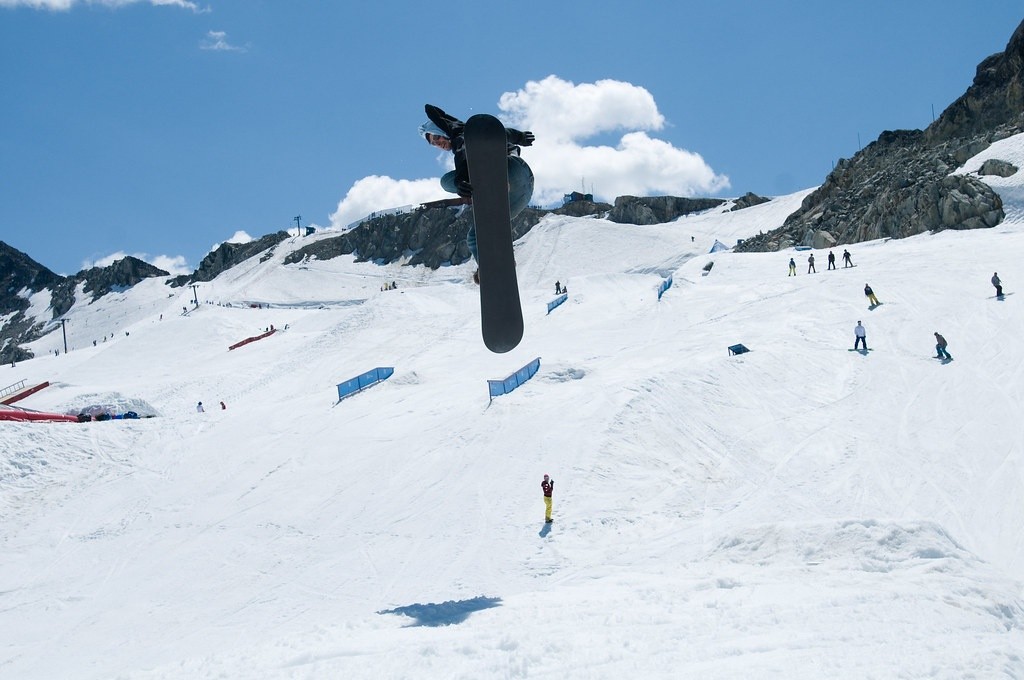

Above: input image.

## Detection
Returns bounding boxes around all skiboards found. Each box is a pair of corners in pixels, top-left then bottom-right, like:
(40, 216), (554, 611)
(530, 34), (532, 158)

(932, 355), (953, 360)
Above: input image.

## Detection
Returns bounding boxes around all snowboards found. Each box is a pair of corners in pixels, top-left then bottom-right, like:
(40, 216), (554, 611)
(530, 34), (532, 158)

(848, 348), (873, 351)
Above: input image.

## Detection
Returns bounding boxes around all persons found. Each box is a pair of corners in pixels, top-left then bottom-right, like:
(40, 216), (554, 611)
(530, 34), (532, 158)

(864, 283), (880, 306)
(933, 332), (951, 360)
(808, 254), (815, 273)
(381, 280), (397, 291)
(842, 249), (853, 267)
(562, 286), (567, 293)
(197, 402), (204, 412)
(541, 474), (555, 524)
(789, 258), (796, 277)
(991, 272), (1004, 296)
(827, 251), (835, 270)
(854, 321), (868, 349)
(417, 103), (534, 286)
(220, 402), (225, 410)
(55, 299), (274, 355)
(554, 280), (561, 294)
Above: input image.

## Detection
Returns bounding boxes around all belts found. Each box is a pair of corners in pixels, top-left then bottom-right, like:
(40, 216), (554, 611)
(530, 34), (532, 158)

(512, 156), (534, 186)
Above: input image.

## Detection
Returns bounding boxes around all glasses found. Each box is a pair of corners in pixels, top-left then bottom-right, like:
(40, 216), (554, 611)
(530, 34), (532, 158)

(431, 134), (441, 147)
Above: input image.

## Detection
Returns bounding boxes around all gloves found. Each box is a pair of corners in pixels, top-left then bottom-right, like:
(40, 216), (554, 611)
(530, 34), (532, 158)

(454, 175), (473, 198)
(519, 131), (535, 146)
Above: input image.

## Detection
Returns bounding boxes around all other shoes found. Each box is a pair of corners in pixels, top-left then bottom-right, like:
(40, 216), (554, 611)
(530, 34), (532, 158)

(473, 273), (480, 285)
(461, 207), (474, 221)
